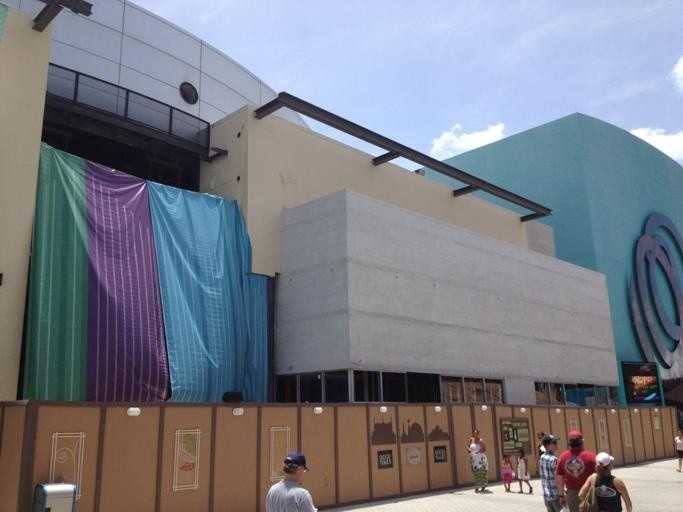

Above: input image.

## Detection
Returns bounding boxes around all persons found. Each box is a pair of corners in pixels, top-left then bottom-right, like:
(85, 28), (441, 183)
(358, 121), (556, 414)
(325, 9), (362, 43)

(673, 429), (683, 472)
(538, 433), (566, 511)
(577, 452), (632, 511)
(262, 452), (319, 512)
(501, 454), (514, 492)
(553, 430), (597, 511)
(536, 431), (546, 456)
(464, 428), (489, 493)
(515, 449), (533, 494)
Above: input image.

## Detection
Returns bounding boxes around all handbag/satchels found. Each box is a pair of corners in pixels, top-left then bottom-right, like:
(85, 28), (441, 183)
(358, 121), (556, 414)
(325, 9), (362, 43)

(579, 472), (598, 512)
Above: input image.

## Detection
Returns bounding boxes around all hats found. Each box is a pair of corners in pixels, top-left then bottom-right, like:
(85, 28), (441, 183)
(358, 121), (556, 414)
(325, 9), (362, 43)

(569, 429), (583, 442)
(283, 453), (310, 472)
(542, 434), (561, 444)
(595, 452), (615, 468)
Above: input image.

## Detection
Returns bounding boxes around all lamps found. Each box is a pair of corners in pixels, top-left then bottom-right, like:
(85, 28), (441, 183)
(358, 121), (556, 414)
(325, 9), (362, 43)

(520, 407), (525, 414)
(611, 409), (616, 414)
(314, 407), (323, 415)
(380, 406), (387, 413)
(555, 408), (560, 413)
(233, 407), (244, 416)
(584, 409), (589, 415)
(127, 406), (140, 417)
(482, 405), (488, 412)
(435, 406), (441, 413)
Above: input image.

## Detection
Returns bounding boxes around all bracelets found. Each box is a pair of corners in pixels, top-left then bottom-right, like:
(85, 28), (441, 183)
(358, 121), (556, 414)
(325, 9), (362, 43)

(558, 494), (564, 497)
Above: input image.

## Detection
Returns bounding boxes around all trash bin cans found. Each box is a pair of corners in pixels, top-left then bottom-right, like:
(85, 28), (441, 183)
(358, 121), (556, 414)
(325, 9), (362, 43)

(32, 482), (77, 512)
